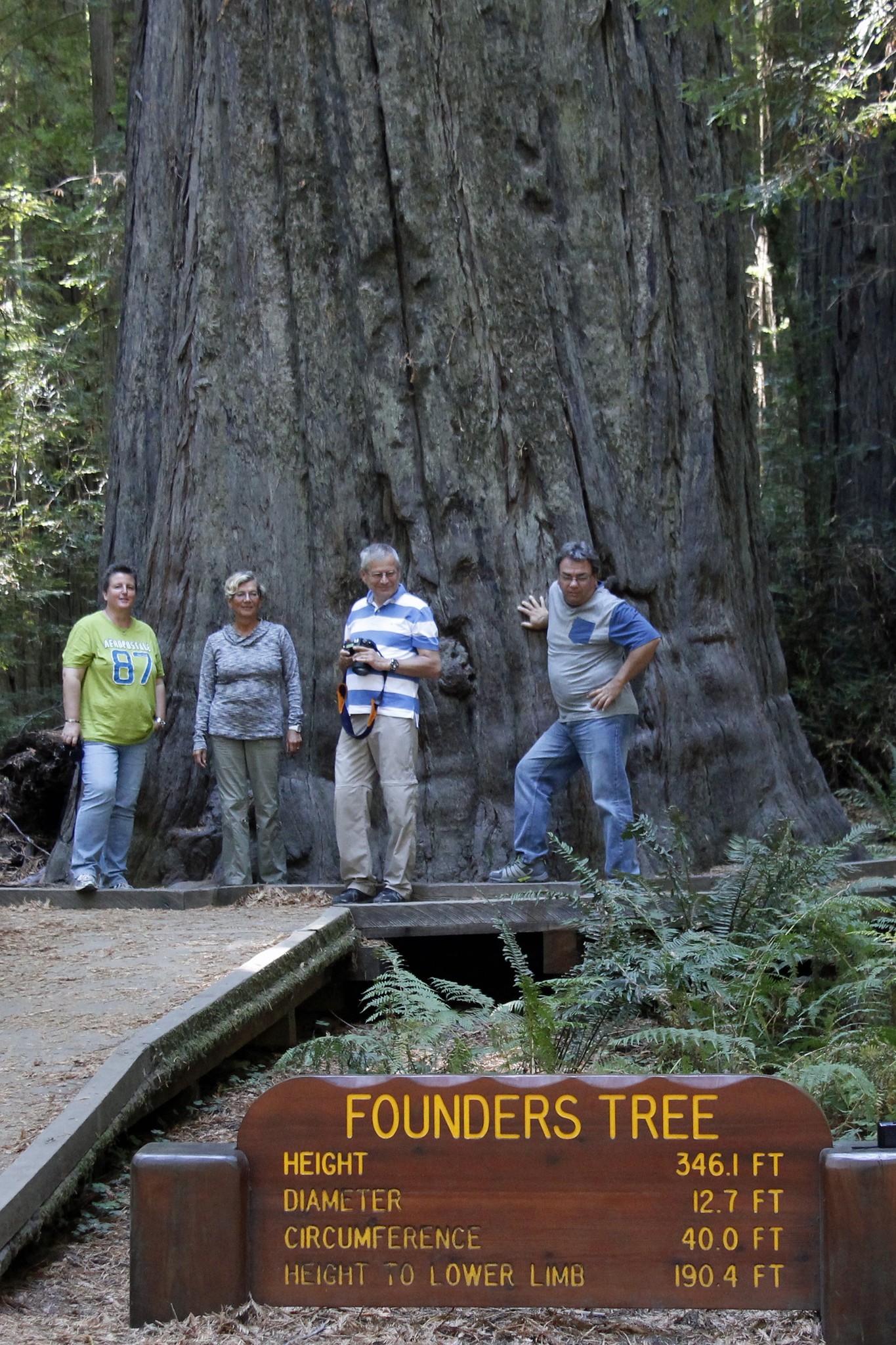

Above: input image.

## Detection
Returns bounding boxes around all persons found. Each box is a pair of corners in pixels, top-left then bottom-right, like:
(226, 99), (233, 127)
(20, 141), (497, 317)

(487, 542), (662, 884)
(335, 543), (442, 903)
(61, 564), (166, 895)
(192, 569), (302, 893)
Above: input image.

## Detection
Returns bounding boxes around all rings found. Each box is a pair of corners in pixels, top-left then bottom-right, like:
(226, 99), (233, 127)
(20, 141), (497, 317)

(297, 747), (299, 749)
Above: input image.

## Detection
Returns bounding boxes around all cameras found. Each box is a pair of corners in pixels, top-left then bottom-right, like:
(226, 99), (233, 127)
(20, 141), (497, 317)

(55, 738), (84, 765)
(342, 638), (376, 676)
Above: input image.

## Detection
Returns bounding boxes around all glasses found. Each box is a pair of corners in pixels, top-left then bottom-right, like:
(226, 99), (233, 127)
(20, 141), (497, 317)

(366, 569), (398, 580)
(558, 572), (592, 584)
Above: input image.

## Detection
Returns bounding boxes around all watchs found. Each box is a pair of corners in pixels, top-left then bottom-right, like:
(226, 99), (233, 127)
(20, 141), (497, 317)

(389, 658), (399, 673)
(153, 716), (165, 728)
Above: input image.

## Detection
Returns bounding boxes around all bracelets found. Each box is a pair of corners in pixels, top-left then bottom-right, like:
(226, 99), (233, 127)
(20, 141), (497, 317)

(65, 719), (80, 724)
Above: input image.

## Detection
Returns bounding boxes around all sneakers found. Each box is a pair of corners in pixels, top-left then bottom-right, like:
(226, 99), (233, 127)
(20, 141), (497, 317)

(488, 855), (549, 882)
(333, 888), (373, 904)
(74, 874), (99, 891)
(373, 888), (405, 903)
(112, 883), (134, 888)
